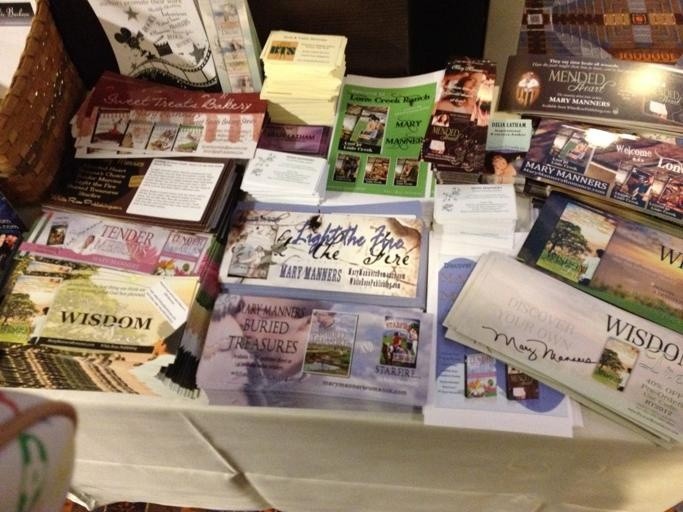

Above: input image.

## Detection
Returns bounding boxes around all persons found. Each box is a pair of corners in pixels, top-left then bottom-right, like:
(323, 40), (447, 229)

(341, 155), (358, 181)
(581, 249), (604, 284)
(384, 328), (417, 364)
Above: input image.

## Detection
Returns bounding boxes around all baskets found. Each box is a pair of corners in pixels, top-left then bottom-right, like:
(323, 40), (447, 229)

(0, 2), (81, 204)
